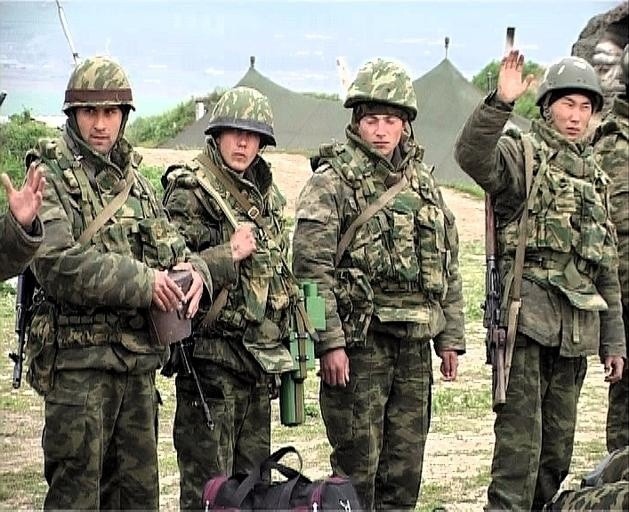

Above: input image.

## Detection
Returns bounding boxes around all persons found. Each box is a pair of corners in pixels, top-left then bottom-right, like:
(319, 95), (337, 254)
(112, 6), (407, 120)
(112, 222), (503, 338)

(161, 87), (295, 512)
(593, 45), (629, 453)
(543, 445), (629, 512)
(292, 60), (466, 512)
(23, 58), (212, 512)
(0, 161), (45, 284)
(454, 49), (626, 512)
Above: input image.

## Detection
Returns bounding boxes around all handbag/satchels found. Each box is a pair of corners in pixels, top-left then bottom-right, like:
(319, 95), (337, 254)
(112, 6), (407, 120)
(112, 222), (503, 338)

(201, 446), (361, 512)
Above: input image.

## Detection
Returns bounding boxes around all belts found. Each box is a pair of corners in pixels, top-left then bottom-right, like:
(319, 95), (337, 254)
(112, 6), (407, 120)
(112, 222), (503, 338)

(524, 247), (601, 282)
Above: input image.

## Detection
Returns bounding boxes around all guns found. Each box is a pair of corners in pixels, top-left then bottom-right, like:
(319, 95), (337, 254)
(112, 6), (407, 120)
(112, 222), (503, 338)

(176, 337), (214, 432)
(9, 271), (33, 388)
(481, 70), (507, 412)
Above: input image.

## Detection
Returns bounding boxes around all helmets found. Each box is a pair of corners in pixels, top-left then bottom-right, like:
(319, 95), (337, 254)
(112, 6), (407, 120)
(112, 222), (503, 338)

(535, 57), (604, 113)
(62, 56), (136, 112)
(205, 86), (276, 146)
(344, 58), (419, 121)
